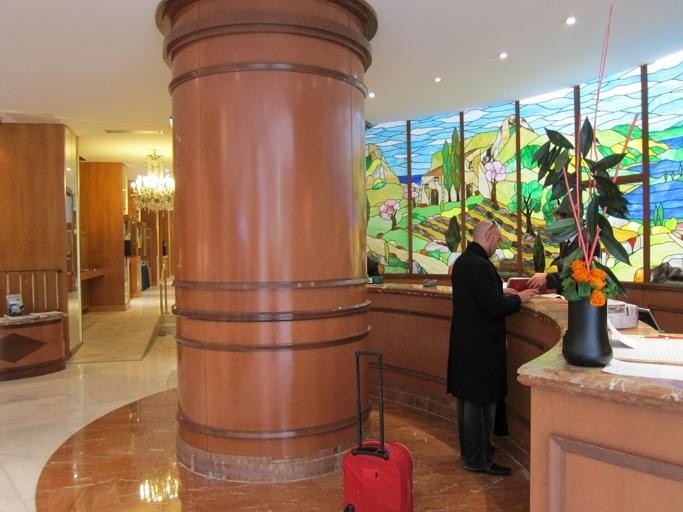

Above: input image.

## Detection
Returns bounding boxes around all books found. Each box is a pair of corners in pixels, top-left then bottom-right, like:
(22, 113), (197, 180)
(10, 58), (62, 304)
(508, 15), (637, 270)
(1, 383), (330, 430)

(502, 277), (531, 292)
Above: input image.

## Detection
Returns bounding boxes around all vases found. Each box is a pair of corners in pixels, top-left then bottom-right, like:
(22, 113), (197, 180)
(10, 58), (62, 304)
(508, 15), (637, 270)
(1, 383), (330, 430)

(561, 284), (614, 369)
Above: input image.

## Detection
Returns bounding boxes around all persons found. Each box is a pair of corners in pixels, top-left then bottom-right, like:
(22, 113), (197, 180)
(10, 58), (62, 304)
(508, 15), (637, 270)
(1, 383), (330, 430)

(447, 222), (539, 476)
(367, 259), (379, 276)
(527, 211), (592, 297)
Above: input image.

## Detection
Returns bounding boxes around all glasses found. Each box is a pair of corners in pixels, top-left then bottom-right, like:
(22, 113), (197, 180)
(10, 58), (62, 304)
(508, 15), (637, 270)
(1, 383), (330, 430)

(485, 219), (497, 236)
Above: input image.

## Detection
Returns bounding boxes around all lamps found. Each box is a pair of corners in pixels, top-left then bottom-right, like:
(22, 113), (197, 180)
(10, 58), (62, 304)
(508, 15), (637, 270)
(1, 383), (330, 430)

(130, 149), (175, 211)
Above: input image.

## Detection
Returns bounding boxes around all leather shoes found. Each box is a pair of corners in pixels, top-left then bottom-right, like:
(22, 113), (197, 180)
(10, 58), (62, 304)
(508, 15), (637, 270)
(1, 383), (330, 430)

(480, 463), (511, 475)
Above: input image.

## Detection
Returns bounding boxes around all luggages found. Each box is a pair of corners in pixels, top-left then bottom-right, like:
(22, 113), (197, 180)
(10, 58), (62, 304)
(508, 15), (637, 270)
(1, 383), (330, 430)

(342, 350), (413, 512)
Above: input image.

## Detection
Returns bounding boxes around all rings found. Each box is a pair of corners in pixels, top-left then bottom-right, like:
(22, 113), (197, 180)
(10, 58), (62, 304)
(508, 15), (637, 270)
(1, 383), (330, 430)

(535, 283), (538, 286)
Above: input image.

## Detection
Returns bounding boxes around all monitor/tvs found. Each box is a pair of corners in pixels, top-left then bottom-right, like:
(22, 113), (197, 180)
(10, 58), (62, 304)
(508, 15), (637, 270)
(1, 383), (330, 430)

(65, 192), (74, 228)
(638, 306), (659, 333)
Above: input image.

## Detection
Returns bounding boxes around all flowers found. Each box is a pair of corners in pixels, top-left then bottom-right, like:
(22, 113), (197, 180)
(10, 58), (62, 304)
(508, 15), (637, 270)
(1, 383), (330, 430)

(532, 117), (631, 308)
